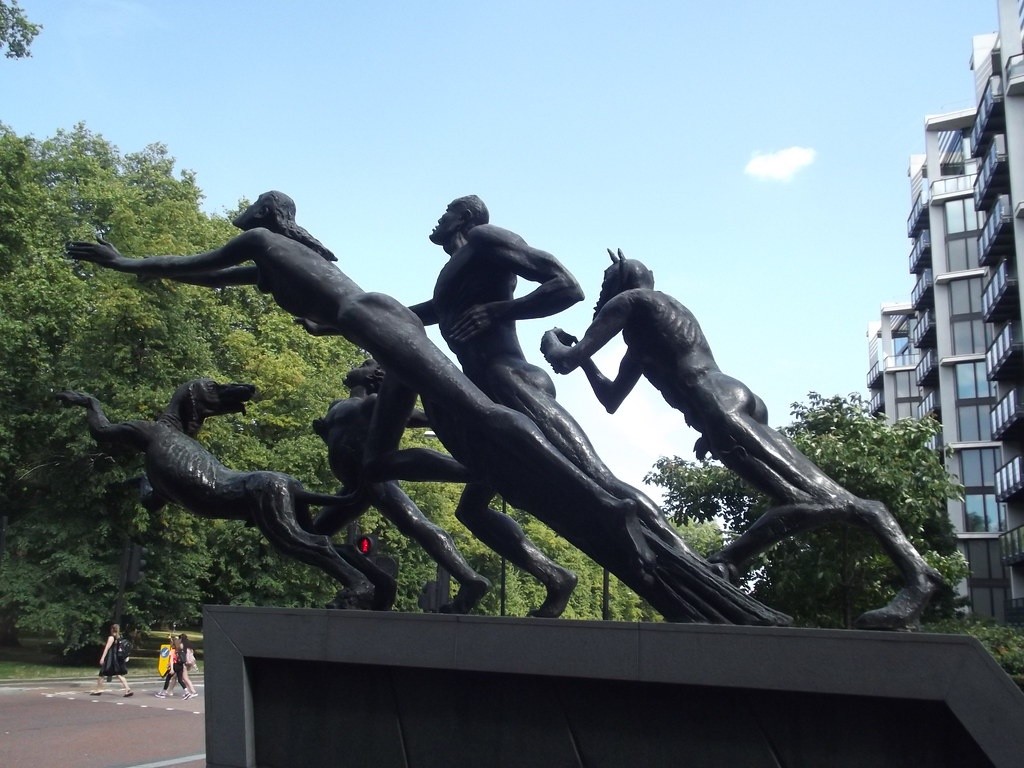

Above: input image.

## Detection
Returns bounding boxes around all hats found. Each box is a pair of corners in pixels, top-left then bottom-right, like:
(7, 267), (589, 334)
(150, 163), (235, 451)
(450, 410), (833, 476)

(179, 634), (187, 639)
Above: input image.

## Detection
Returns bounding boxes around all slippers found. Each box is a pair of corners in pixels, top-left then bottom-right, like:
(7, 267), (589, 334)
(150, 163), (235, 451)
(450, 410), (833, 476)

(90, 692), (102, 696)
(123, 692), (133, 697)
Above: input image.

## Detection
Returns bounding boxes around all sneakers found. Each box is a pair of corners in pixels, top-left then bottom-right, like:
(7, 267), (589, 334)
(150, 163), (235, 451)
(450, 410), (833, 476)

(155, 692), (165, 699)
(190, 692), (199, 698)
(166, 690), (173, 696)
(182, 693), (190, 700)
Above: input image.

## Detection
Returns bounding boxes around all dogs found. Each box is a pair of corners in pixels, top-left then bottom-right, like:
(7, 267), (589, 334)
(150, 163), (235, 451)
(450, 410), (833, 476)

(42, 377), (400, 612)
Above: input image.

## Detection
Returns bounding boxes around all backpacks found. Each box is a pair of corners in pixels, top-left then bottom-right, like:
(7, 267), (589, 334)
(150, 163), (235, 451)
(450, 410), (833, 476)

(114, 633), (133, 661)
(186, 648), (199, 672)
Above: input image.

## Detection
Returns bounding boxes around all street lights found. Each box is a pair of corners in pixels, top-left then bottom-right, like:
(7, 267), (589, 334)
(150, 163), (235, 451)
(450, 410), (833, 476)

(424, 431), (506, 614)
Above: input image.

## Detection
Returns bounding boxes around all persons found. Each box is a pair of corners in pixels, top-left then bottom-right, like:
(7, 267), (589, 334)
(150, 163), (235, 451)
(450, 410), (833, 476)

(68, 189), (948, 625)
(90, 623), (133, 697)
(155, 633), (198, 700)
